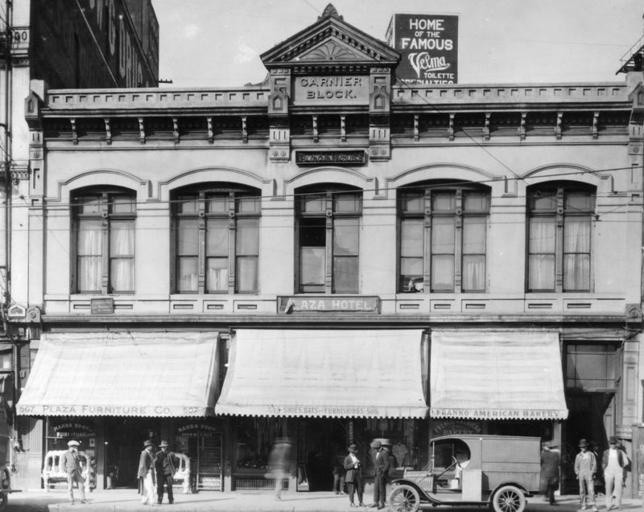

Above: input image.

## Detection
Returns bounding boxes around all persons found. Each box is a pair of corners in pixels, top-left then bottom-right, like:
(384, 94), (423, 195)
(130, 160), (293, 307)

(150, 440), (178, 505)
(616, 436), (632, 488)
(600, 435), (626, 511)
(538, 439), (564, 507)
(59, 439), (88, 504)
(368, 441), (388, 510)
(343, 443), (364, 507)
(328, 441), (347, 495)
(136, 439), (155, 507)
(573, 438), (598, 512)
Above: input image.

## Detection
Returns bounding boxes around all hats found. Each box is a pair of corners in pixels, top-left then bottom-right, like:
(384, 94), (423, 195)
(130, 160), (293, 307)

(143, 439), (169, 449)
(348, 439), (381, 455)
(67, 440), (81, 448)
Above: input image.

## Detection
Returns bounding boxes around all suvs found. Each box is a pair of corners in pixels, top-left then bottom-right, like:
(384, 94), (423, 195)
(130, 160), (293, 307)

(387, 433), (542, 512)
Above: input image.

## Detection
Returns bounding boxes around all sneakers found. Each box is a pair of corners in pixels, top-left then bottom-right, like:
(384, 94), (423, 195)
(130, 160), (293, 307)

(141, 498), (174, 506)
(350, 502), (386, 510)
(68, 500), (90, 506)
(334, 491), (345, 496)
